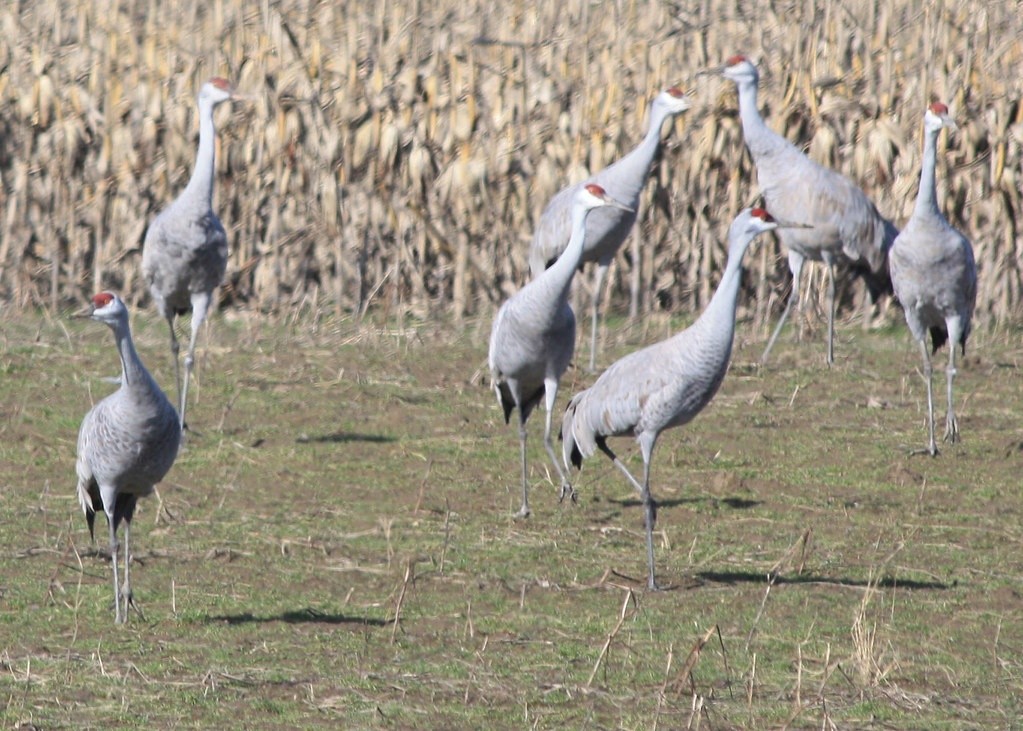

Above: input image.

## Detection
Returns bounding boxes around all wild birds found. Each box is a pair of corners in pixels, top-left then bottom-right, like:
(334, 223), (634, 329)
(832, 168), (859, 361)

(526, 87), (712, 372)
(556, 208), (815, 592)
(67, 289), (182, 624)
(887, 101), (978, 461)
(141, 78), (253, 436)
(489, 183), (638, 522)
(696, 55), (900, 369)
